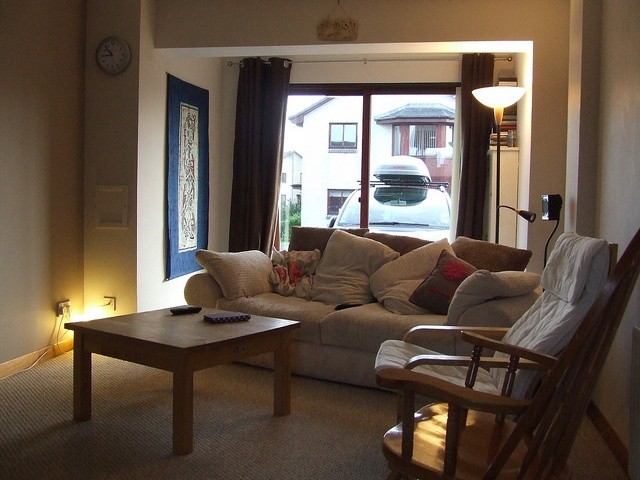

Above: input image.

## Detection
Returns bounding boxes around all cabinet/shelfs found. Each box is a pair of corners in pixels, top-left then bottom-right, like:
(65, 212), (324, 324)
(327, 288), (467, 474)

(487, 144), (519, 248)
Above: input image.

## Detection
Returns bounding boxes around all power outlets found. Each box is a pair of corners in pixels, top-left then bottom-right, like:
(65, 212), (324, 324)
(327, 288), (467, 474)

(57, 300), (71, 317)
(103, 298), (116, 311)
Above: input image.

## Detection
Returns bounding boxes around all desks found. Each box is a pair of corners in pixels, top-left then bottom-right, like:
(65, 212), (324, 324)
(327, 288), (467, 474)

(64, 304), (301, 456)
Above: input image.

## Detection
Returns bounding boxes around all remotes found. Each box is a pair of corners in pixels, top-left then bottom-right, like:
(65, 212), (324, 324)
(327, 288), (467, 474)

(169, 304), (203, 316)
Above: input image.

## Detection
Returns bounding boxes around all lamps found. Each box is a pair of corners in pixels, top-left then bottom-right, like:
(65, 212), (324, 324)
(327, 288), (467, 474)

(472, 85), (527, 126)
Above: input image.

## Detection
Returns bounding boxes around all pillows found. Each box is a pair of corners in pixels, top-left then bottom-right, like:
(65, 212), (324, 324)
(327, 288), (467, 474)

(270, 247), (321, 300)
(369, 238), (457, 317)
(442, 269), (540, 327)
(450, 235), (533, 271)
(288, 228), (369, 251)
(195, 248), (275, 301)
(408, 249), (480, 316)
(310, 229), (401, 310)
(363, 230), (435, 256)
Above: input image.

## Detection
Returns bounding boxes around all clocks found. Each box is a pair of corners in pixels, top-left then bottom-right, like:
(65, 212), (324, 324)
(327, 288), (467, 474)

(94, 36), (132, 75)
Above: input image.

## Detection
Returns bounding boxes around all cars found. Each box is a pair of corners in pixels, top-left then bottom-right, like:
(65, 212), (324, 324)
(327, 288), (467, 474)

(330, 185), (451, 243)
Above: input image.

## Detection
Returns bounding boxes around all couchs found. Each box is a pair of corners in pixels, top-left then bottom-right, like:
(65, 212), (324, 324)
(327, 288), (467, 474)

(184, 227), (540, 406)
